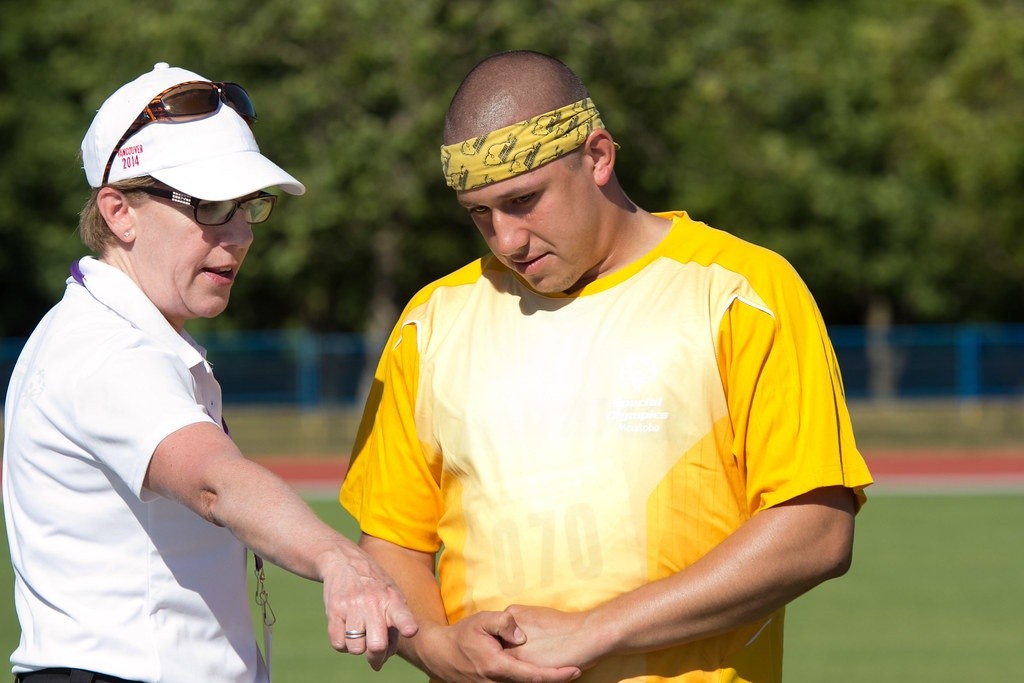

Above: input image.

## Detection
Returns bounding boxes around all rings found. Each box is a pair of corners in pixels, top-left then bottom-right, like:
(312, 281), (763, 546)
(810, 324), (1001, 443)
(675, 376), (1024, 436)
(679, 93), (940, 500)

(344, 630), (367, 641)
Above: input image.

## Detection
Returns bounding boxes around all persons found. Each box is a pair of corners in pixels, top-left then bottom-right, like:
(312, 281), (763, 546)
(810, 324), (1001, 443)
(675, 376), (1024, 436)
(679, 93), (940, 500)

(338, 50), (876, 683)
(3, 60), (426, 683)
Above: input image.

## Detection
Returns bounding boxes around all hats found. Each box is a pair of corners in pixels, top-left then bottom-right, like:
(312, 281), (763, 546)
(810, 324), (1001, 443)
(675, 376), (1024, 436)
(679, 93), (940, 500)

(81, 60), (307, 204)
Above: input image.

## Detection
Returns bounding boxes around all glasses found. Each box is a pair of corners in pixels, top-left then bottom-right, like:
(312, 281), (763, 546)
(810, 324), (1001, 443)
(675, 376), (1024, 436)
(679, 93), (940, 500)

(132, 184), (280, 226)
(101, 79), (260, 185)
